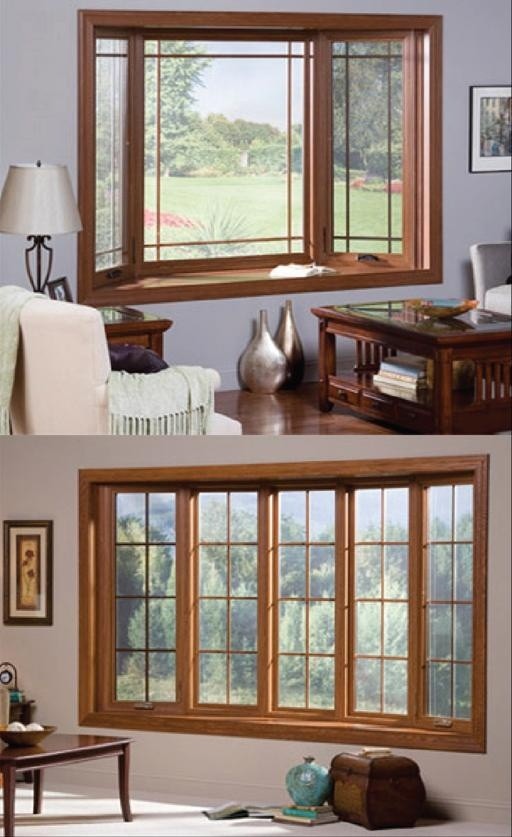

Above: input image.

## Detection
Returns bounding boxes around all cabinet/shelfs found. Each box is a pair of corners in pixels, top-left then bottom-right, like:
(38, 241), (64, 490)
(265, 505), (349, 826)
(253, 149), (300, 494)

(94, 304), (174, 372)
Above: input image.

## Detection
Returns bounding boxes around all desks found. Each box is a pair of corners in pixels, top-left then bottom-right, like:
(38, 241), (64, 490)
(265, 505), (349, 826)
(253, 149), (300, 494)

(1, 730), (135, 835)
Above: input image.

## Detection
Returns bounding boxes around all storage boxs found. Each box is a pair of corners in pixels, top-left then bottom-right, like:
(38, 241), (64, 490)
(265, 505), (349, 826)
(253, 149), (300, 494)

(326, 752), (426, 830)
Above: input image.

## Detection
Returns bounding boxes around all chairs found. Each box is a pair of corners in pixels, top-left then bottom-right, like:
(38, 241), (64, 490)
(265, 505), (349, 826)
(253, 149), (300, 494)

(0, 285), (243, 435)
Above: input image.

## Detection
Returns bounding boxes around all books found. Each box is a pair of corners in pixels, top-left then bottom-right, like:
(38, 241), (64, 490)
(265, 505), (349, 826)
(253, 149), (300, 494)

(269, 262), (336, 278)
(372, 354), (428, 404)
(201, 801), (340, 827)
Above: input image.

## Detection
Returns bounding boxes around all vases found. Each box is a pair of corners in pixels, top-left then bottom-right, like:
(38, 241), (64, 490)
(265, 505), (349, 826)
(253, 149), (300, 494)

(236, 297), (305, 395)
(285, 757), (329, 805)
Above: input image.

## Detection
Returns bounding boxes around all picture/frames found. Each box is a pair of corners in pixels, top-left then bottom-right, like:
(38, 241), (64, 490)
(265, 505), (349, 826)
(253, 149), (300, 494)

(467, 83), (512, 172)
(44, 276), (74, 303)
(3, 517), (55, 628)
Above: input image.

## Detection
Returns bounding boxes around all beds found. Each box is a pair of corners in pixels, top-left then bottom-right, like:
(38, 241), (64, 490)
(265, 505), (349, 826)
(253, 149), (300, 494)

(310, 297), (511, 435)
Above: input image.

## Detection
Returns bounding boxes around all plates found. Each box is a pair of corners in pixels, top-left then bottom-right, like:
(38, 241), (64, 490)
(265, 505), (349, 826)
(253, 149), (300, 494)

(405, 298), (479, 319)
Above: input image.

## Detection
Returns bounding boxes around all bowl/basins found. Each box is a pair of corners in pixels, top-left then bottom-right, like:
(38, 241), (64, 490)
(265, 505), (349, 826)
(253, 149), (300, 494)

(0, 722), (58, 747)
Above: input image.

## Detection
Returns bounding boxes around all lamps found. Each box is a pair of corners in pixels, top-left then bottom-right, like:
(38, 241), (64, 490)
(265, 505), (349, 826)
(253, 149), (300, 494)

(0, 161), (82, 295)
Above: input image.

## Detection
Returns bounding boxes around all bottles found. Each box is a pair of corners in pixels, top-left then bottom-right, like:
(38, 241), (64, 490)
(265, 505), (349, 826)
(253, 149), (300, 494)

(0, 686), (9, 733)
(284, 754), (334, 805)
